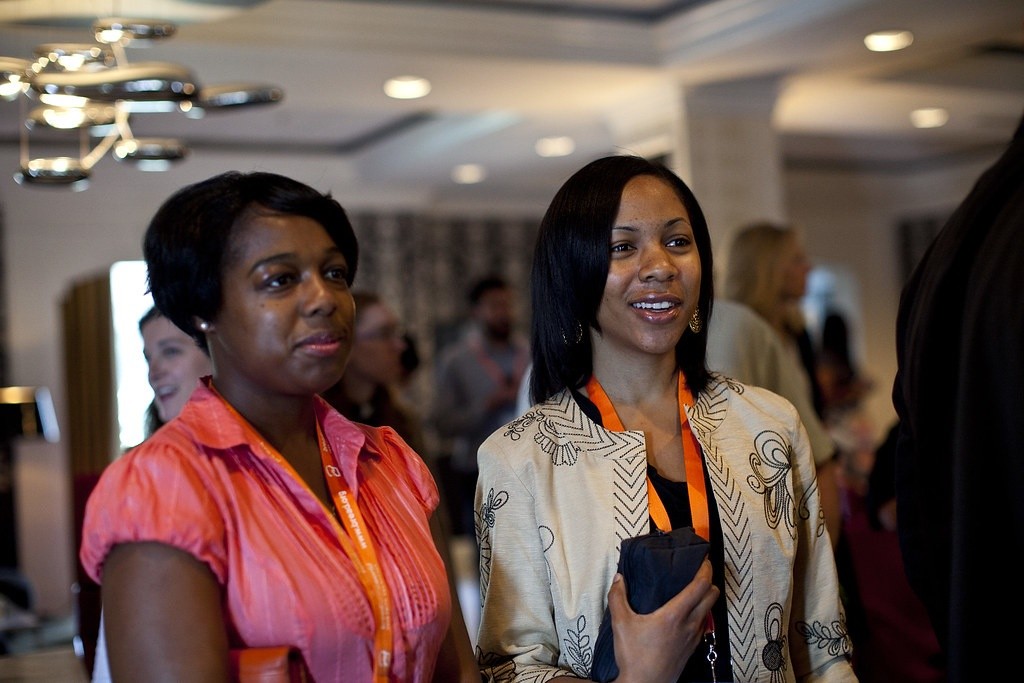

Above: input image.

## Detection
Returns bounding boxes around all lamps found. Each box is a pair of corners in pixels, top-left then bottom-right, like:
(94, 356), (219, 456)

(0, 0), (284, 193)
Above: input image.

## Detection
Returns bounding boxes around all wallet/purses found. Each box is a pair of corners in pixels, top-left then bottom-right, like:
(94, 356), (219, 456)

(592, 528), (710, 683)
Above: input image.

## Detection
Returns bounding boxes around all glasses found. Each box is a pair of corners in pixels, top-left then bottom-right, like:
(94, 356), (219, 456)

(355, 323), (406, 340)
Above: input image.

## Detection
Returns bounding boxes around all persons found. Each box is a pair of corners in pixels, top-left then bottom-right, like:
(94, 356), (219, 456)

(474, 156), (862, 683)
(865, 108), (1024, 683)
(324, 292), (426, 455)
(432, 276), (524, 530)
(82, 173), (483, 683)
(706, 223), (871, 549)
(139, 304), (212, 441)
